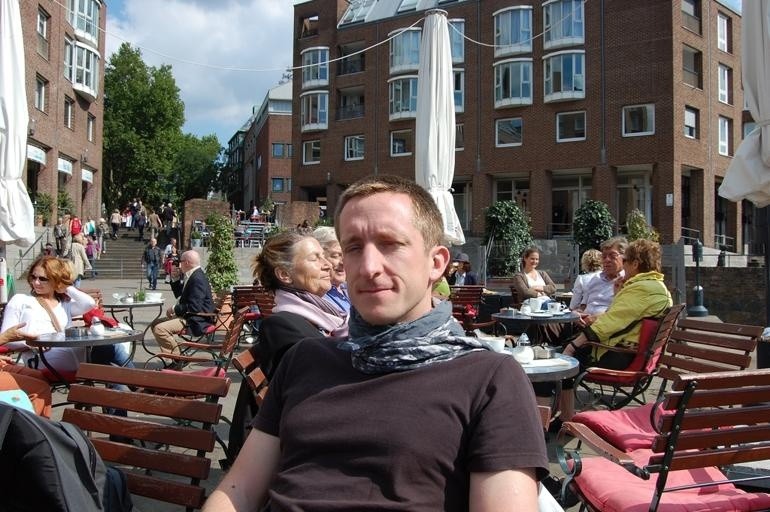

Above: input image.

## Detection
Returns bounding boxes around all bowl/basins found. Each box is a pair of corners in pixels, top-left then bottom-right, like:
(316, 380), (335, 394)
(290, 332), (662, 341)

(145, 292), (162, 300)
(500, 308), (518, 316)
(481, 337), (506, 354)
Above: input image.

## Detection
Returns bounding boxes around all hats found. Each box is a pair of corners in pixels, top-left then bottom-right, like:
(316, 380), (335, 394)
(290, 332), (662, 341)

(453, 253), (469, 263)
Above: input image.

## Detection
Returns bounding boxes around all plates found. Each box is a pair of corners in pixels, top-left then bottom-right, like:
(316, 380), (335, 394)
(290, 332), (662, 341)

(529, 313), (565, 318)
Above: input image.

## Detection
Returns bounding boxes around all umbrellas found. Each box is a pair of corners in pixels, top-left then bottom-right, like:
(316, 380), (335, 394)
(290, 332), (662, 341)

(415, 8), (467, 246)
(0, 0), (36, 246)
(717, 1), (770, 210)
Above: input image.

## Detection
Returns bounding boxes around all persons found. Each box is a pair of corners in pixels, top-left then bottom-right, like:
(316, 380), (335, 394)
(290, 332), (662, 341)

(562, 236), (629, 370)
(142, 238), (178, 289)
(203, 172), (549, 512)
(109, 198), (147, 242)
(512, 249), (563, 343)
(312, 226), (351, 314)
(560, 249), (603, 341)
(148, 202), (177, 241)
(0, 255), (138, 448)
(532, 239), (674, 442)
(244, 232), (350, 371)
(443, 254), (478, 286)
(53, 214), (109, 287)
(152, 250), (214, 369)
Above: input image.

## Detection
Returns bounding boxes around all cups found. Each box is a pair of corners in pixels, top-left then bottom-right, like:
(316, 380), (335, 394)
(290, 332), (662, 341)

(547, 303), (562, 313)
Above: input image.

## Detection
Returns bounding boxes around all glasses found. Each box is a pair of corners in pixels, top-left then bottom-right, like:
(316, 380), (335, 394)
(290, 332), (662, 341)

(28, 273), (49, 283)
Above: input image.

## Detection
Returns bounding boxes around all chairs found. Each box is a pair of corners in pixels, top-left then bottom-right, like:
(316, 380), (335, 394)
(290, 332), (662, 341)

(0, 283), (770, 512)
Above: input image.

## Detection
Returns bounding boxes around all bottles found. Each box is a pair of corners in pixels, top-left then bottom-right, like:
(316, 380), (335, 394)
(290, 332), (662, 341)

(138, 289), (145, 301)
(250, 300), (259, 313)
(520, 301), (531, 315)
(126, 292), (137, 303)
(512, 332), (535, 365)
(89, 316), (105, 335)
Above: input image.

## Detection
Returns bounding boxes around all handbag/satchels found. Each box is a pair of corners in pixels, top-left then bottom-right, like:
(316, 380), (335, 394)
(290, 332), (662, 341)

(0, 401), (137, 511)
